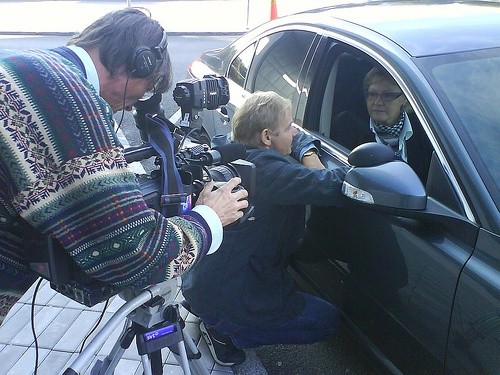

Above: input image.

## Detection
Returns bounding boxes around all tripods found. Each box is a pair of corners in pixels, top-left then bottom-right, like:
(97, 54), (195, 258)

(62, 284), (210, 375)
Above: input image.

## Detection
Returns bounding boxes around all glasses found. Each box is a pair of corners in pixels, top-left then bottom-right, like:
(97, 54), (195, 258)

(365, 91), (404, 103)
(138, 80), (157, 102)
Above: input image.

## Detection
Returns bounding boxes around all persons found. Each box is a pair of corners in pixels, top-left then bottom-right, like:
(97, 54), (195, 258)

(0, 5), (250, 326)
(179, 89), (353, 367)
(339, 65), (430, 164)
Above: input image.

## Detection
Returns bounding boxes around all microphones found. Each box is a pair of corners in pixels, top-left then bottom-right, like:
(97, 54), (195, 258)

(200, 142), (246, 166)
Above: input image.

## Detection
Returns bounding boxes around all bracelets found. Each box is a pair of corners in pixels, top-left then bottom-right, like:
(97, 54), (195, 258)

(304, 150), (315, 156)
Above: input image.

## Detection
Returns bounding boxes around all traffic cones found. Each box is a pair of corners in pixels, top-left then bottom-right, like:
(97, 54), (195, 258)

(271, 0), (276, 21)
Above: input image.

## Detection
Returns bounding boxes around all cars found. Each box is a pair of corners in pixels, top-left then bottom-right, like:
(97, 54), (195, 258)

(163, 0), (500, 375)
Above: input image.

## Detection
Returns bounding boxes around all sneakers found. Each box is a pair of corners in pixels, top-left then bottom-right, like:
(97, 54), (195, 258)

(199, 321), (246, 367)
(180, 300), (204, 323)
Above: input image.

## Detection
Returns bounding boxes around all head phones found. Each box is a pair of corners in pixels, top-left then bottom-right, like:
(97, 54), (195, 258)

(128, 31), (168, 78)
